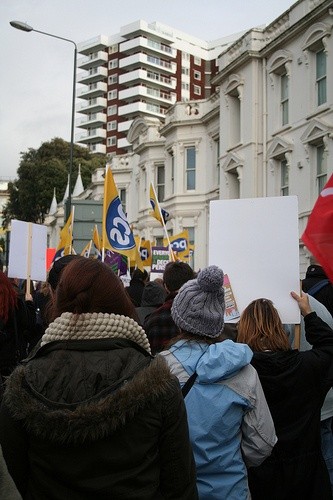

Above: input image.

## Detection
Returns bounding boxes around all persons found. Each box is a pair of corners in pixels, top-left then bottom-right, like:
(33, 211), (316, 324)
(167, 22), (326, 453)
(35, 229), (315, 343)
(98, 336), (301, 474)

(0, 254), (333, 348)
(153, 264), (278, 500)
(290, 290), (333, 500)
(234, 288), (333, 499)
(1, 257), (201, 500)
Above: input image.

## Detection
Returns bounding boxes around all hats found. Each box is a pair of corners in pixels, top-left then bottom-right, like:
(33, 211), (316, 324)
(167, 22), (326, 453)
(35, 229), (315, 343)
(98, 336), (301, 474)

(141, 281), (166, 305)
(170, 265), (226, 338)
(305, 265), (323, 275)
(47, 255), (81, 288)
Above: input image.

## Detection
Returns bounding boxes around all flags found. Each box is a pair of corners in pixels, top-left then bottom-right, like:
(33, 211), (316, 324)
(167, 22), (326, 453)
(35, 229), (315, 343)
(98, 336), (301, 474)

(1, 162), (195, 281)
(300, 171), (333, 284)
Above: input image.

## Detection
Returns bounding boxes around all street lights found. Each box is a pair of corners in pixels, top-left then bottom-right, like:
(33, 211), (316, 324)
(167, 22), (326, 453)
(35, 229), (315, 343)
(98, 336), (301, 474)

(9, 20), (77, 216)
